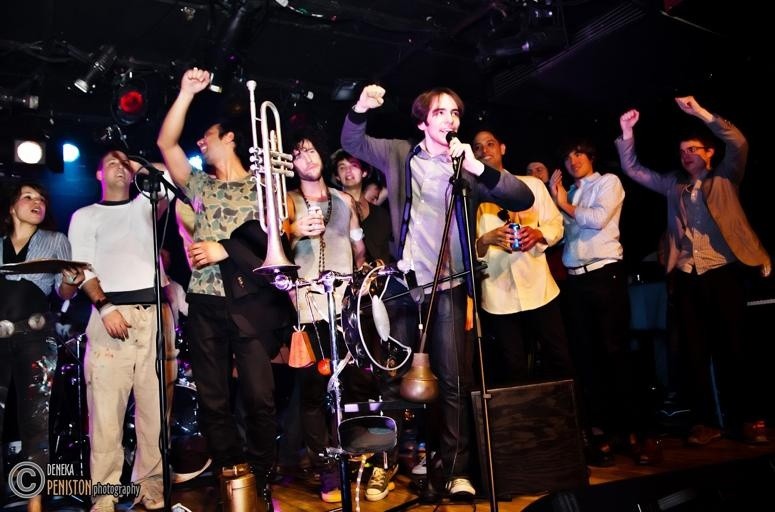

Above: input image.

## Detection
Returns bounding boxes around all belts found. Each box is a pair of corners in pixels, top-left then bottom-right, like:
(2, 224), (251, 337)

(566, 257), (619, 278)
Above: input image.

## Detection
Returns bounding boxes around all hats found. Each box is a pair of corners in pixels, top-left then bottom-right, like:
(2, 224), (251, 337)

(326, 146), (376, 188)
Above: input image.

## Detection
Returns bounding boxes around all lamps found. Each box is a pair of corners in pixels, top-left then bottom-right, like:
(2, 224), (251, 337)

(62, 143), (81, 163)
(13, 137), (49, 167)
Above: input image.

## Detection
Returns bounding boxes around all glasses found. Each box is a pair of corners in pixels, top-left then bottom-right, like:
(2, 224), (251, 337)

(676, 144), (712, 157)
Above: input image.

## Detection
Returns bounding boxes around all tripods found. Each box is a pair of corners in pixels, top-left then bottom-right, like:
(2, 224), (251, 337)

(50, 334), (95, 503)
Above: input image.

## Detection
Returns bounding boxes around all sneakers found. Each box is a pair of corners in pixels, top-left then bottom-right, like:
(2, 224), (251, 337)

(742, 421), (769, 445)
(89, 493), (120, 512)
(366, 460), (401, 501)
(320, 466), (343, 504)
(688, 425), (721, 445)
(446, 475), (477, 500)
(410, 449), (441, 475)
(133, 476), (165, 509)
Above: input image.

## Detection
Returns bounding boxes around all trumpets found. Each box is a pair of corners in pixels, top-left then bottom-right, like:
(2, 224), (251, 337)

(242, 74), (302, 277)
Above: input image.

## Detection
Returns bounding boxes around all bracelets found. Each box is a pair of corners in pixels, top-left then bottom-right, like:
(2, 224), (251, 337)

(94, 299), (110, 309)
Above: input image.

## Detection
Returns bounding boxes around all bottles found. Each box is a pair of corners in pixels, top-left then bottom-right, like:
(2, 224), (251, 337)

(310, 207), (324, 234)
(508, 222), (521, 253)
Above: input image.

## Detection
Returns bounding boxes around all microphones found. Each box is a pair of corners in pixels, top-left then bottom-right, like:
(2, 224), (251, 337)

(445, 130), (465, 188)
(96, 118), (122, 148)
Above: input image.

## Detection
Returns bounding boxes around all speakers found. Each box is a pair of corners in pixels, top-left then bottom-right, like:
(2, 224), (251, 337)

(470, 377), (591, 500)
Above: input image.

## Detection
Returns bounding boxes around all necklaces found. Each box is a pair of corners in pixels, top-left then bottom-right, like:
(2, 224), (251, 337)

(297, 182), (333, 279)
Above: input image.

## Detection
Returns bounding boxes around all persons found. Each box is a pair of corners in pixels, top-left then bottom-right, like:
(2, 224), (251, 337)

(362, 177), (383, 204)
(474, 129), (564, 382)
(284, 133), (386, 503)
(338, 85), (536, 503)
(69, 149), (179, 512)
(329, 148), (391, 275)
(0, 181), (87, 320)
(549, 140), (652, 466)
(613, 96), (772, 445)
(524, 154), (553, 188)
(157, 64), (283, 512)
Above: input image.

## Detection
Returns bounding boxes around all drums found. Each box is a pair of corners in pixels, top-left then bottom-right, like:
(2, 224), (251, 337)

(123, 383), (213, 484)
